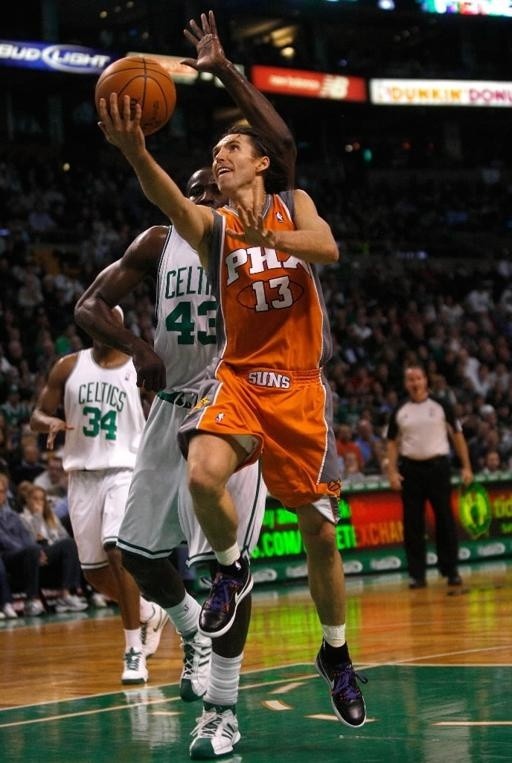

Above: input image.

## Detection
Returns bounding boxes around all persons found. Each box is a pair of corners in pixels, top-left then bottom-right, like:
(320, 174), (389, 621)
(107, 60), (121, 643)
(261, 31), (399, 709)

(96, 93), (367, 728)
(74, 12), (297, 759)
(1, 0), (512, 619)
(30, 304), (167, 682)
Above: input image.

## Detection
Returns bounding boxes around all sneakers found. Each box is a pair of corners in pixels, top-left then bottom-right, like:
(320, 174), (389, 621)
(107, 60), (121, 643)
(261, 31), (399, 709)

(139, 603), (168, 657)
(0, 594), (109, 620)
(448, 575), (462, 585)
(199, 556), (254, 638)
(189, 701), (241, 759)
(409, 581), (425, 588)
(122, 647), (148, 684)
(315, 637), (369, 729)
(176, 626), (212, 701)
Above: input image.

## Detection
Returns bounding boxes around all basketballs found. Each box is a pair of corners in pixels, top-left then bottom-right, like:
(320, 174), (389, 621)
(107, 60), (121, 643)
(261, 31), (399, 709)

(95, 57), (176, 136)
